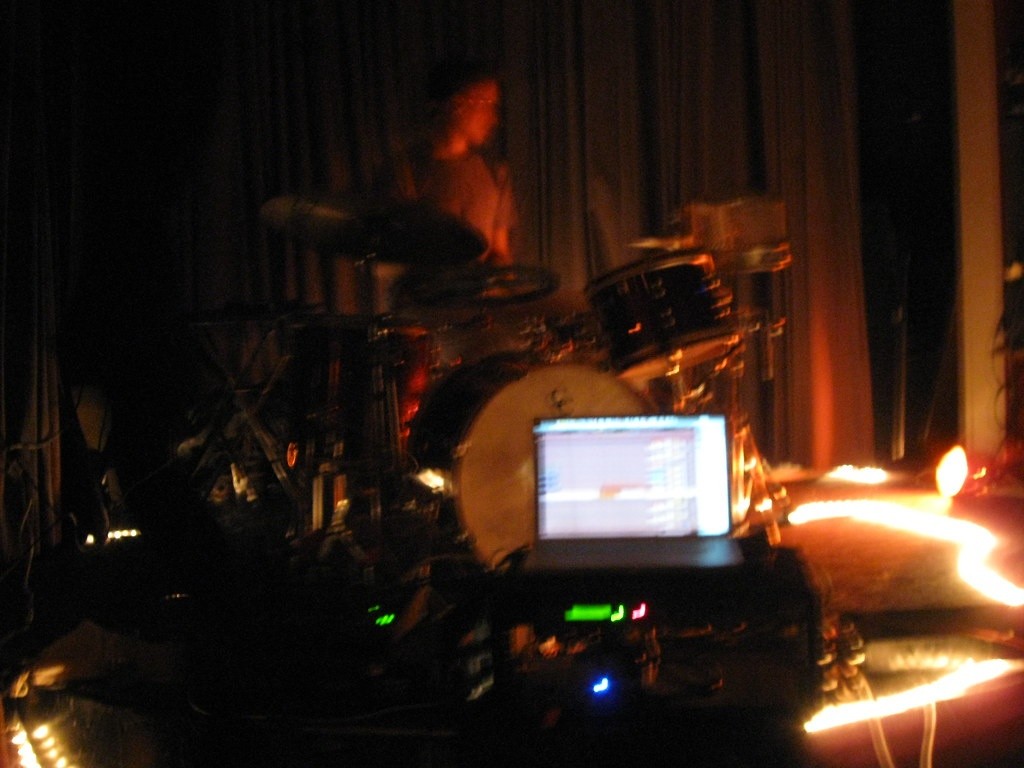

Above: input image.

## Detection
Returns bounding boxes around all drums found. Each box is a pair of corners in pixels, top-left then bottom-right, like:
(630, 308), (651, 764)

(402, 345), (654, 582)
(583, 247), (744, 383)
(280, 309), (437, 455)
(729, 236), (797, 337)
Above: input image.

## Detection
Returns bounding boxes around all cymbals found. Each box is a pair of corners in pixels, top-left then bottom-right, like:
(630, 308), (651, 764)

(256, 171), (492, 271)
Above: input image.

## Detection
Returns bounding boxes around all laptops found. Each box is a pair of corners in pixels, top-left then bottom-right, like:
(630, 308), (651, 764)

(523, 415), (745, 577)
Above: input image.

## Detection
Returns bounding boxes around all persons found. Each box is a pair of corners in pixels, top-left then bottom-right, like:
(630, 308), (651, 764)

(395, 67), (520, 441)
